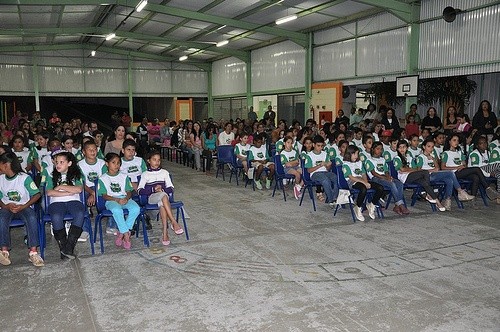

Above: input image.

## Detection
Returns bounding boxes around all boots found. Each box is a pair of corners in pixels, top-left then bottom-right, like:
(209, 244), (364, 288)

(53, 225), (83, 262)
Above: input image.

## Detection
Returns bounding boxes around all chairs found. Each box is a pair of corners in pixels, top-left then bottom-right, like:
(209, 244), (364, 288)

(0, 128), (500, 260)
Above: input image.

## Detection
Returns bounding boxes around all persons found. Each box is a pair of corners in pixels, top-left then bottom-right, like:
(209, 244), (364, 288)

(0, 100), (500, 267)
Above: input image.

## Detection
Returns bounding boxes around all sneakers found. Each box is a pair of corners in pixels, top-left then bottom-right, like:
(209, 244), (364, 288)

(353, 205), (365, 221)
(366, 202), (379, 219)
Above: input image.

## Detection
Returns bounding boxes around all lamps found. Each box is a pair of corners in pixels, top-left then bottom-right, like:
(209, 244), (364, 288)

(136, 0), (148, 13)
(275, 14), (298, 24)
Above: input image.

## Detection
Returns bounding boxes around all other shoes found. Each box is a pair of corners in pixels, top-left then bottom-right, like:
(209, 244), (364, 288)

(245, 173), (251, 185)
(162, 239), (171, 246)
(443, 199), (451, 211)
(196, 167), (201, 172)
(293, 184), (302, 200)
(106, 227), (118, 235)
(206, 170), (212, 176)
(171, 224), (184, 235)
(435, 204), (446, 212)
(486, 187), (500, 201)
(467, 200), (480, 210)
(329, 201), (342, 212)
(0, 250), (12, 266)
(393, 205), (410, 216)
(425, 196), (437, 204)
(254, 178), (262, 190)
(77, 231), (90, 242)
(457, 190), (475, 201)
(28, 250), (45, 266)
(115, 231), (131, 249)
(265, 177), (272, 190)
